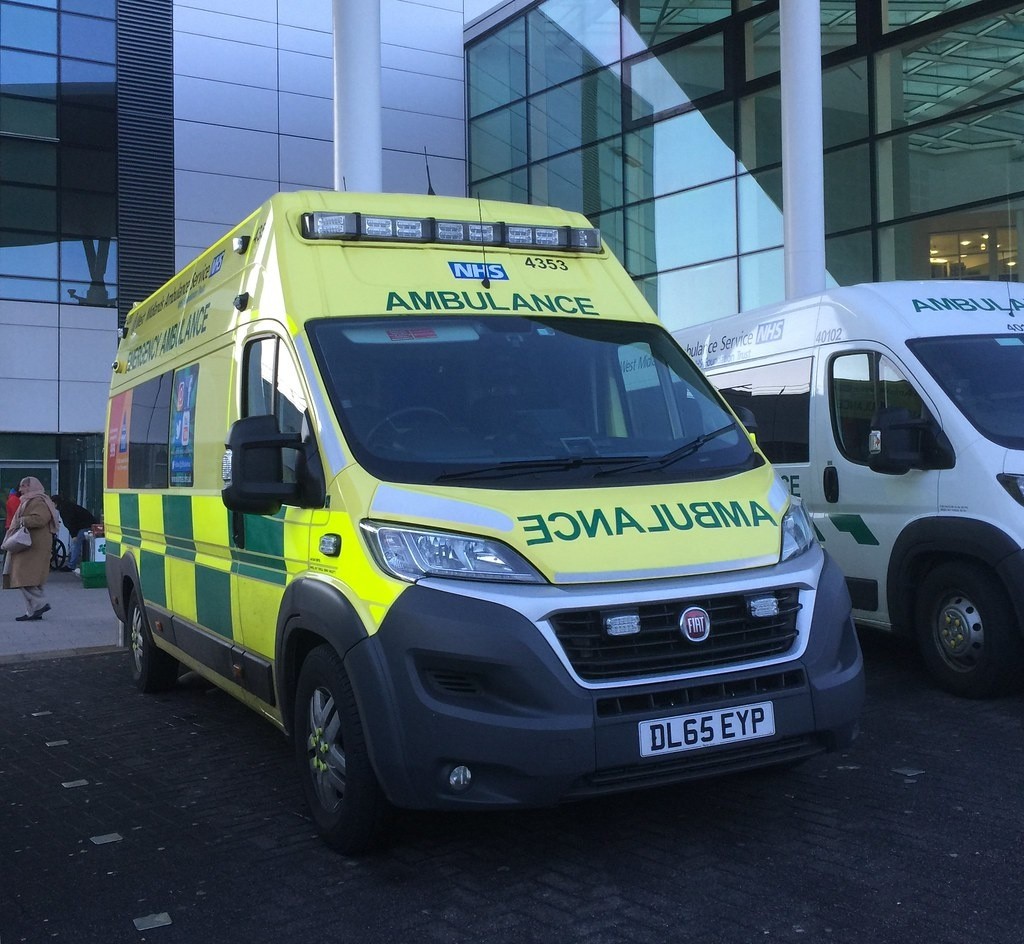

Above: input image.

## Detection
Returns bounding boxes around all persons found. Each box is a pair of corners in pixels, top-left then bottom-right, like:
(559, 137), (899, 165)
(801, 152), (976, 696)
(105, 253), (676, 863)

(51, 495), (95, 572)
(3, 483), (21, 566)
(0, 477), (60, 621)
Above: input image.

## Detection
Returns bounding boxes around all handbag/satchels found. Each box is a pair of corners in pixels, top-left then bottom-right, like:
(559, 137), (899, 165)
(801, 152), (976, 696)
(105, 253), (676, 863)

(1, 522), (32, 552)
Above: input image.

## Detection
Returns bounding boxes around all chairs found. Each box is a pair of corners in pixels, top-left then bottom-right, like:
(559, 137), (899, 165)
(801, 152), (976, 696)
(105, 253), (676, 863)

(868, 407), (918, 471)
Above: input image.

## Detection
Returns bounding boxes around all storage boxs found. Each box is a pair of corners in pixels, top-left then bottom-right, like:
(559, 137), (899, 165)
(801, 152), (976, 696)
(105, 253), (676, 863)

(89, 537), (106, 563)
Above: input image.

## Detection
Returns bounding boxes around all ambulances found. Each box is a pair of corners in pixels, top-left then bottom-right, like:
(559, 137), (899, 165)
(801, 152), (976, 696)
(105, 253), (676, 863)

(617, 253), (1024, 711)
(102, 148), (866, 860)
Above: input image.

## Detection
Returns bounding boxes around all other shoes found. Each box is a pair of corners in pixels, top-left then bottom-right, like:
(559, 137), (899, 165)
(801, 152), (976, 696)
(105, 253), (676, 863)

(60, 566), (75, 572)
(30, 604), (50, 619)
(16, 614), (42, 621)
